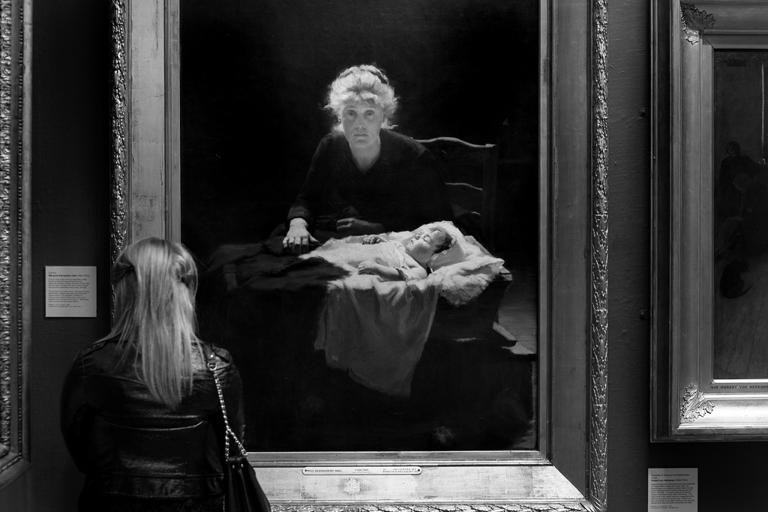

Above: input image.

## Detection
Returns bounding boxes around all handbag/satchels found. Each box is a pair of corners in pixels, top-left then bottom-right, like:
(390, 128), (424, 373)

(225, 457), (271, 512)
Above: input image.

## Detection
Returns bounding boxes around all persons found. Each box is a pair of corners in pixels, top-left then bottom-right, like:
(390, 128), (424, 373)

(283, 65), (454, 256)
(61, 237), (247, 510)
(358, 226), (464, 280)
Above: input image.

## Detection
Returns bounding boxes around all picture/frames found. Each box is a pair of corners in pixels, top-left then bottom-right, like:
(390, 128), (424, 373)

(109, 0), (608, 511)
(647, 3), (768, 447)
